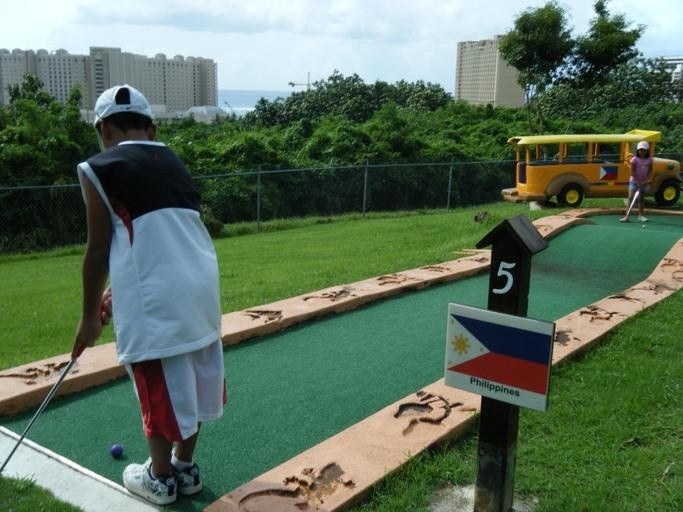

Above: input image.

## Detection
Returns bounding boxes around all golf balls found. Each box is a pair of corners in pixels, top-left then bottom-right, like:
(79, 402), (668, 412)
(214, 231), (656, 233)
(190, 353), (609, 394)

(110, 445), (123, 458)
(642, 225), (645, 228)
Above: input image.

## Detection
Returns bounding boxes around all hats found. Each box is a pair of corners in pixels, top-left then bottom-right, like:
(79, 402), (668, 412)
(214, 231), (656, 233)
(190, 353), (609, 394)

(93, 83), (153, 150)
(636, 141), (649, 150)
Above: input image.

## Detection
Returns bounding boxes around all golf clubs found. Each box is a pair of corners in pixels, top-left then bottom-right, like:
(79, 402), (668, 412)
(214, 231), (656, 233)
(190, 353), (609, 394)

(620, 188), (640, 222)
(1, 292), (112, 474)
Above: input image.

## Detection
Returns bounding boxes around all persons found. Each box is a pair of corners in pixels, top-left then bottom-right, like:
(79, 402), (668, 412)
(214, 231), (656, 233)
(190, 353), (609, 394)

(624, 140), (654, 223)
(69, 82), (227, 505)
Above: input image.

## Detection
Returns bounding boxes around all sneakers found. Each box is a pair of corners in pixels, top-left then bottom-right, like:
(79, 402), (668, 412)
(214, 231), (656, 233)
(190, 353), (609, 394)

(122, 457), (178, 506)
(171, 460), (202, 497)
(638, 216), (648, 222)
